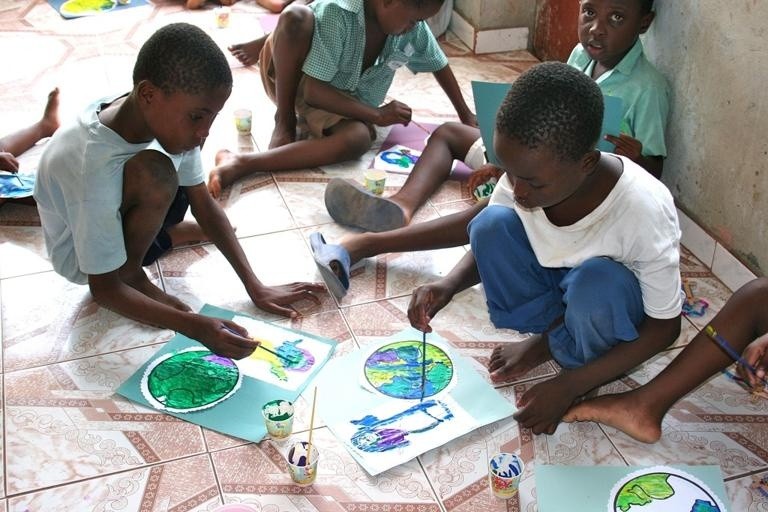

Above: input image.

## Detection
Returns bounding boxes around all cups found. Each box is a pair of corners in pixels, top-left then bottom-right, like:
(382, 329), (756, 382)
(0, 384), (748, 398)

(260, 399), (297, 443)
(282, 441), (321, 486)
(363, 165), (388, 198)
(229, 107), (253, 136)
(488, 451), (524, 503)
(213, 4), (229, 28)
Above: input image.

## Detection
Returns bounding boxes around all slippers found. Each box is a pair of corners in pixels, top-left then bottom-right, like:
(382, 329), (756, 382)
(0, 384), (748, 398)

(323, 176), (405, 232)
(308, 233), (352, 299)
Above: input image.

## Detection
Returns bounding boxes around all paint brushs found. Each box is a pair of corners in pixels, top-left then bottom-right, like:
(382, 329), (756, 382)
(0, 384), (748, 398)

(383, 99), (432, 135)
(1, 149), (25, 188)
(705, 324), (767, 386)
(223, 325), (299, 365)
(420, 327), (427, 406)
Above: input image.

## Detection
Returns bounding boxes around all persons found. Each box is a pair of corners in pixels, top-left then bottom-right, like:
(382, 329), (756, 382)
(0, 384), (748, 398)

(36, 23), (326, 359)
(310, 0), (671, 303)
(0, 88), (61, 174)
(408, 61), (683, 436)
(560, 277), (768, 444)
(156, 1), (293, 65)
(208, 0), (478, 198)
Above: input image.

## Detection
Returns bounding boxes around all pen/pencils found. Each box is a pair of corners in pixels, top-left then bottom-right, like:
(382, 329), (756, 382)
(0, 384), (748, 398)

(306, 387), (318, 463)
